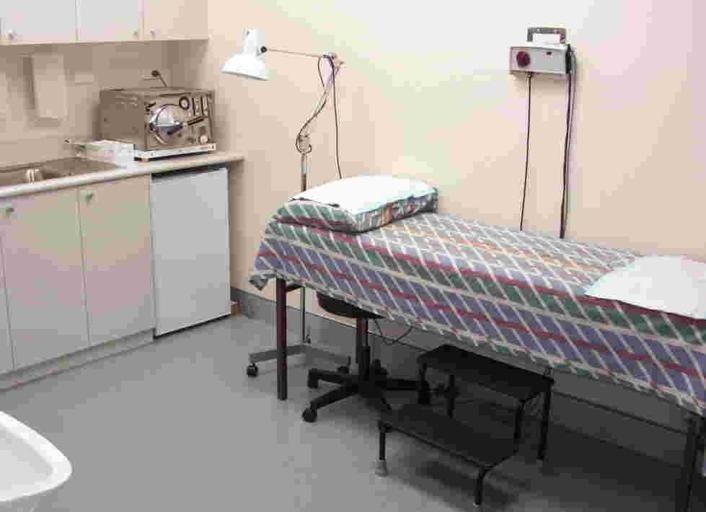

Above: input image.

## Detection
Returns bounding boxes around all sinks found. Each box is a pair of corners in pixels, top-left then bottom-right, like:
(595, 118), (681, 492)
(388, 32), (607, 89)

(0, 410), (74, 512)
(0, 164), (66, 187)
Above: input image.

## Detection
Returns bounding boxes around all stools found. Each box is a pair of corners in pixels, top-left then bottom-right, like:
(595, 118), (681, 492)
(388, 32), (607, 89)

(301, 291), (431, 423)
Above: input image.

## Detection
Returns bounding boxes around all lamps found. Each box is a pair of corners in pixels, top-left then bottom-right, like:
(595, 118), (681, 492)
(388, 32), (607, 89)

(220, 26), (352, 377)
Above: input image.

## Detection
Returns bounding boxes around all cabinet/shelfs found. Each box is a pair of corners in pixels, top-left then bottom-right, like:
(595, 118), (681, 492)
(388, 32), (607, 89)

(1, 2), (209, 48)
(0, 171), (154, 392)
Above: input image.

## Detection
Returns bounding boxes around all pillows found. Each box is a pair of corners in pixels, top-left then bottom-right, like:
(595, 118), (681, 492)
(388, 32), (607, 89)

(273, 174), (439, 233)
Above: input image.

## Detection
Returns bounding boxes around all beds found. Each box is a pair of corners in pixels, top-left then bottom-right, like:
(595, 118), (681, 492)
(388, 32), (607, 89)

(267, 213), (705, 511)
